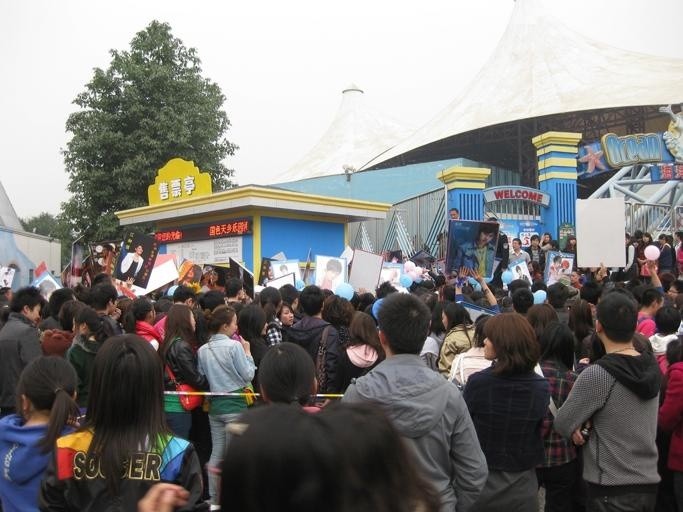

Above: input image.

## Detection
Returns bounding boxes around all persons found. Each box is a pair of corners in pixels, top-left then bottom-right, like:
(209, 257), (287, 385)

(0, 272), (489, 510)
(206, 268), (225, 293)
(317, 259), (342, 291)
(183, 264), (202, 290)
(457, 230), (683, 512)
(97, 245), (115, 271)
(450, 209), (461, 220)
(451, 223), (498, 278)
(262, 263), (275, 286)
(119, 243), (144, 281)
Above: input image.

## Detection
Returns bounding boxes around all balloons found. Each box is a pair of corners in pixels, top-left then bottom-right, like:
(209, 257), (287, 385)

(404, 261), (416, 271)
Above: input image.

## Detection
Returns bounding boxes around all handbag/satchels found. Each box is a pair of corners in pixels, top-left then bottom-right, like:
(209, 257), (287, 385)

(313, 362), (324, 392)
(176, 383), (205, 409)
